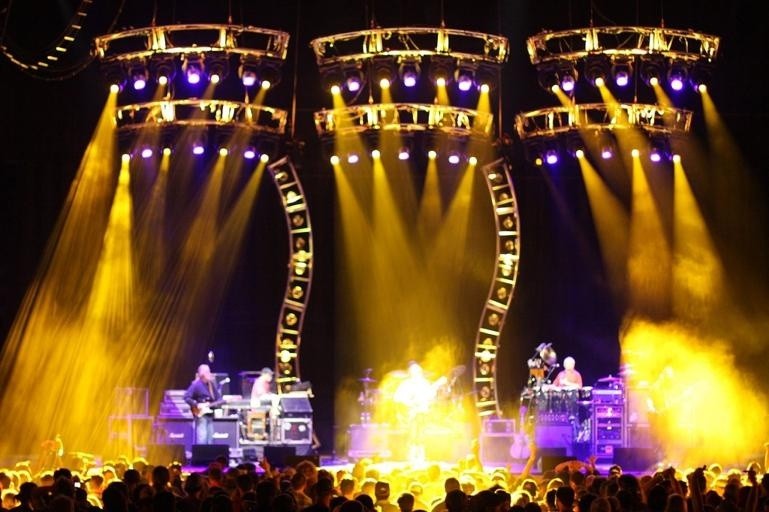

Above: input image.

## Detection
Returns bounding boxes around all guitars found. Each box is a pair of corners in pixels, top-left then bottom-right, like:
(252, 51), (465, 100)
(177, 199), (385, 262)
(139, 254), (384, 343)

(510, 387), (541, 460)
(191, 395), (243, 419)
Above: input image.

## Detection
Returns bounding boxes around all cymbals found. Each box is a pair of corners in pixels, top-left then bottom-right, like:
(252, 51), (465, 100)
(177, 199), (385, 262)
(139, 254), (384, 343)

(357, 377), (374, 383)
(598, 376), (621, 383)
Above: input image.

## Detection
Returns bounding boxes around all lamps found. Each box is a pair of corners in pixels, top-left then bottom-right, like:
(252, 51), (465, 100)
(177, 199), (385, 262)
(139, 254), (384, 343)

(109, 59), (274, 168)
(326, 61), (497, 168)
(534, 58), (709, 166)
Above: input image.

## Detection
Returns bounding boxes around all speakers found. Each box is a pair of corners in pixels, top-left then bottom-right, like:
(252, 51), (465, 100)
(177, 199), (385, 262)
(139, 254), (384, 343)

(191, 445), (229, 466)
(264, 446), (319, 468)
(145, 443), (186, 466)
(538, 456), (578, 472)
(612, 448), (658, 471)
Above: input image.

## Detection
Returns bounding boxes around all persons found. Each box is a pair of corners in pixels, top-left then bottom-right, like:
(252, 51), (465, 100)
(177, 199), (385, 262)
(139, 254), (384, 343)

(250, 367), (278, 406)
(183, 364), (224, 444)
(1, 436), (769, 512)
(551, 357), (583, 388)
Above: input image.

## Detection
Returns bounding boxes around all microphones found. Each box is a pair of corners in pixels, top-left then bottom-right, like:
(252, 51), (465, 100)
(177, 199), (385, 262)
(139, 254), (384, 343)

(551, 363), (560, 367)
(219, 377), (230, 384)
